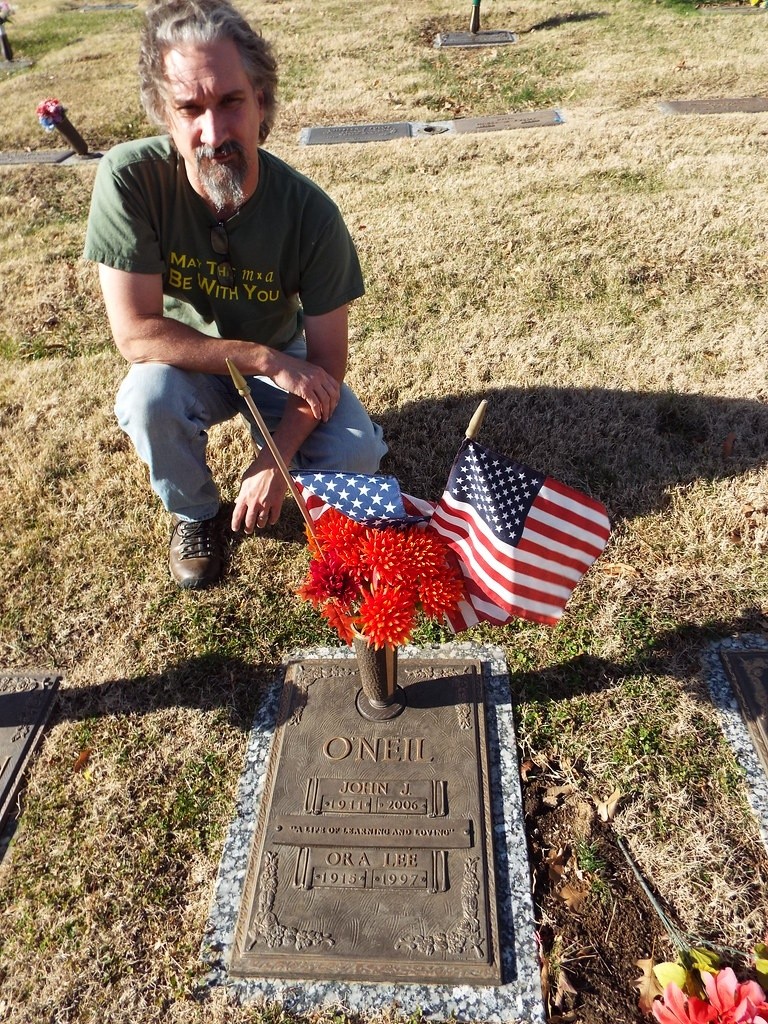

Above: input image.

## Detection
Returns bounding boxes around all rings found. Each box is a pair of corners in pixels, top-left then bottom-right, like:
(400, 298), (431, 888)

(258, 515), (269, 521)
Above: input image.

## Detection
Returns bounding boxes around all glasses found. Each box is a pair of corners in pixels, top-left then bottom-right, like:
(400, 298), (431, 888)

(210, 220), (235, 289)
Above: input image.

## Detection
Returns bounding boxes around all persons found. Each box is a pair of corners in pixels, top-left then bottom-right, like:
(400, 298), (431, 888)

(82, 2), (389, 593)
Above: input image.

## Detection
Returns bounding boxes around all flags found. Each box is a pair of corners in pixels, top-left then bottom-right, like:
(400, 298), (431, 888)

(287, 466), (437, 539)
(423, 438), (611, 637)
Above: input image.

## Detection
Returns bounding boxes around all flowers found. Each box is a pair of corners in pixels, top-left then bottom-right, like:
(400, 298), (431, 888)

(617, 838), (768, 1024)
(301, 507), (464, 651)
(0, 2), (19, 26)
(36, 98), (68, 131)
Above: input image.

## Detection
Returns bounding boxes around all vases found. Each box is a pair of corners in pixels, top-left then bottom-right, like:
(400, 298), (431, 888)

(55, 117), (88, 157)
(0, 28), (14, 62)
(350, 624), (400, 710)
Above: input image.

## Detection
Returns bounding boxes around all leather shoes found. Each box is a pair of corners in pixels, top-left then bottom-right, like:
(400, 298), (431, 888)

(167, 518), (224, 589)
